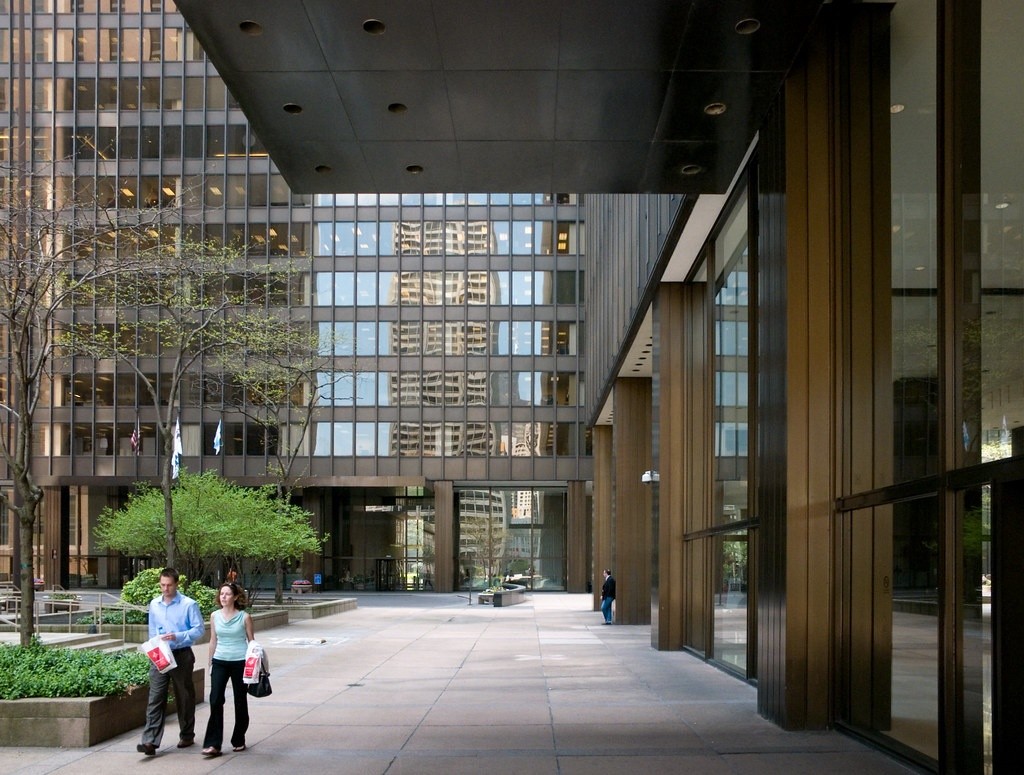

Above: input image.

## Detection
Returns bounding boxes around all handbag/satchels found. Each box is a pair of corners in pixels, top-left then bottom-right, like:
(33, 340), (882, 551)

(243, 648), (273, 697)
(142, 635), (177, 674)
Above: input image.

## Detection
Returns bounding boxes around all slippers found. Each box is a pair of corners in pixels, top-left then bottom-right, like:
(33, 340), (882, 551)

(232, 744), (245, 752)
(201, 746), (223, 756)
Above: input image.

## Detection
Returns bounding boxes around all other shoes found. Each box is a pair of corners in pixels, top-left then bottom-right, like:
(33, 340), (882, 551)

(602, 622), (611, 625)
(137, 743), (156, 755)
(177, 737), (194, 748)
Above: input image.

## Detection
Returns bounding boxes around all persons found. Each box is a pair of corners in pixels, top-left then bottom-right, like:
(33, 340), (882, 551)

(136, 567), (206, 756)
(423, 569), (433, 589)
(600, 568), (616, 625)
(200, 583), (255, 755)
